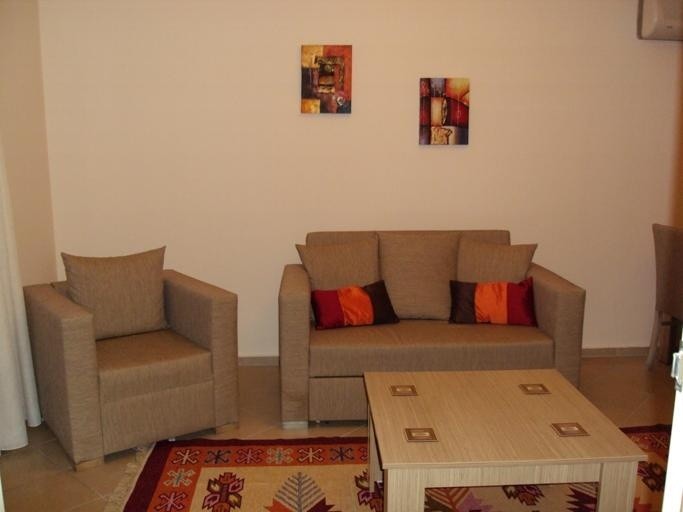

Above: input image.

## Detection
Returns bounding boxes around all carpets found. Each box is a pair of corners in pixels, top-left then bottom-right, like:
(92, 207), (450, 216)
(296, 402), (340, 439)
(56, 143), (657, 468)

(102, 421), (673, 511)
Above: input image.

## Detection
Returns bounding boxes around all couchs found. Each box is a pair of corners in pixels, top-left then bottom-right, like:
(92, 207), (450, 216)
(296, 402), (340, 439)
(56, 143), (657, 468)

(272, 229), (591, 432)
(22, 267), (243, 470)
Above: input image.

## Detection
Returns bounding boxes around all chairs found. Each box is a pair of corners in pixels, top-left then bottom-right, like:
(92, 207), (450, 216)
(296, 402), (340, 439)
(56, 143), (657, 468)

(646, 219), (682, 384)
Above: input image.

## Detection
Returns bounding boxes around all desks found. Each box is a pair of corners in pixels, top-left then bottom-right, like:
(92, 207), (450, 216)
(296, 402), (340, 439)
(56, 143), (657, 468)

(352, 360), (651, 511)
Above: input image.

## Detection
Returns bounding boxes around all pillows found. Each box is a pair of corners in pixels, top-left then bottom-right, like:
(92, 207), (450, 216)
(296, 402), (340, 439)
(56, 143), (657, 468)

(57, 246), (170, 341)
(456, 240), (538, 284)
(444, 276), (537, 327)
(378, 227), (460, 323)
(292, 234), (379, 293)
(309, 280), (400, 331)
